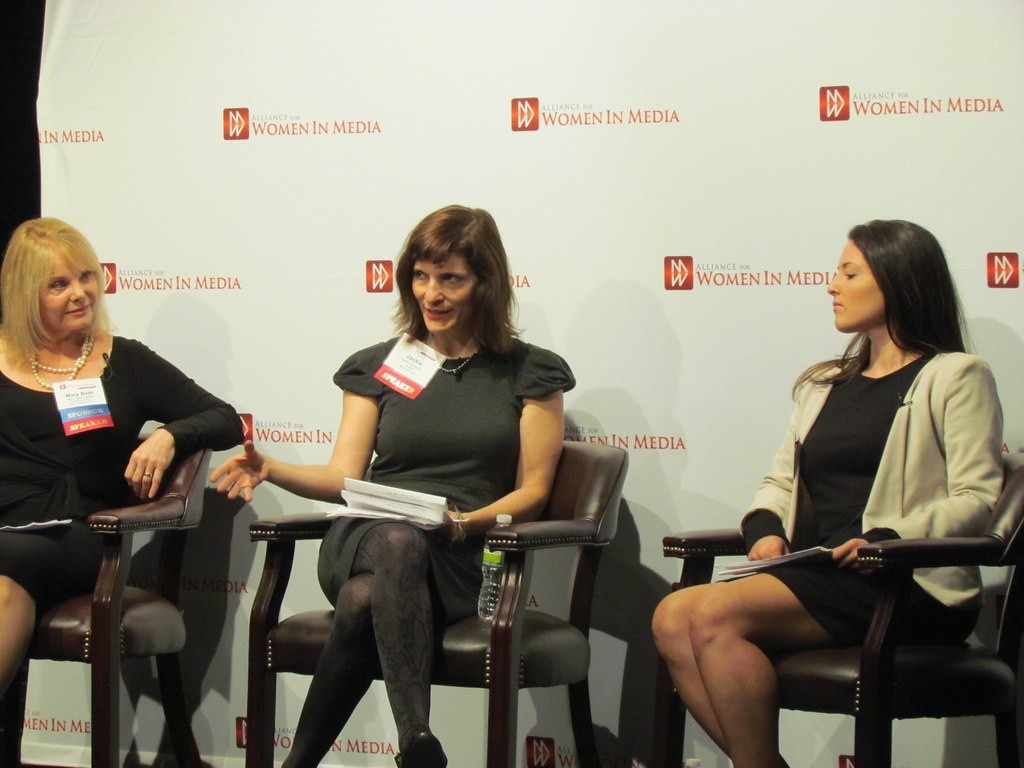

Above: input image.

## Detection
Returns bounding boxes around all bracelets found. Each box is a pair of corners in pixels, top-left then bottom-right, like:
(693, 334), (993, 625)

(451, 510), (466, 542)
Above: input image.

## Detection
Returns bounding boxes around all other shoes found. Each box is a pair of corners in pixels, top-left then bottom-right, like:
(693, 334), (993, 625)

(395, 730), (447, 768)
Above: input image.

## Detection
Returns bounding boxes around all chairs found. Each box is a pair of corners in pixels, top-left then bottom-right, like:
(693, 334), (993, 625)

(0, 422), (214, 768)
(652, 451), (1024, 768)
(245, 441), (629, 768)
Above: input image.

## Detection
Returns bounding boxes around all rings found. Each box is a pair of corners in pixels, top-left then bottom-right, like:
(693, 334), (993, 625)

(144, 473), (152, 477)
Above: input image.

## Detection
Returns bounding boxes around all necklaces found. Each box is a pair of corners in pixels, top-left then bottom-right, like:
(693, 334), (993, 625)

(27, 333), (94, 389)
(438, 351), (476, 372)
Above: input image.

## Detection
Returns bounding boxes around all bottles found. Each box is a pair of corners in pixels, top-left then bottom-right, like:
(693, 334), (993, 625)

(478, 515), (512, 620)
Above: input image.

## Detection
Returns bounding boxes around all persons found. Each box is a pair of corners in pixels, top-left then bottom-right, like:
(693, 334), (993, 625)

(651, 219), (1004, 768)
(0, 219), (243, 697)
(211, 205), (577, 768)
